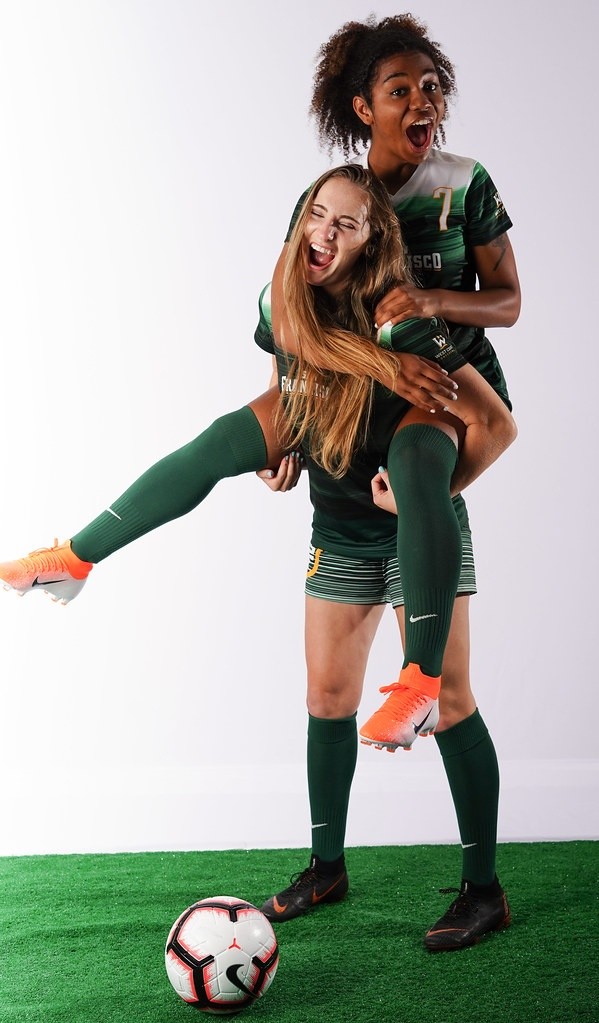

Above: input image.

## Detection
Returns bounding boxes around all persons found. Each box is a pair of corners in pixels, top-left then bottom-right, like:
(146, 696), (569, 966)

(257, 164), (517, 952)
(0, 9), (521, 754)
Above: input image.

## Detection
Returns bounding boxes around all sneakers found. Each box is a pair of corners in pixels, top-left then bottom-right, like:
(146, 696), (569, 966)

(360, 663), (443, 753)
(0, 537), (93, 605)
(424, 873), (512, 952)
(260, 854), (348, 922)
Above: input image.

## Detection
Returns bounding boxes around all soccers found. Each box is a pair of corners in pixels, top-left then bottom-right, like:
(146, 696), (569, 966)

(165, 895), (278, 1014)
